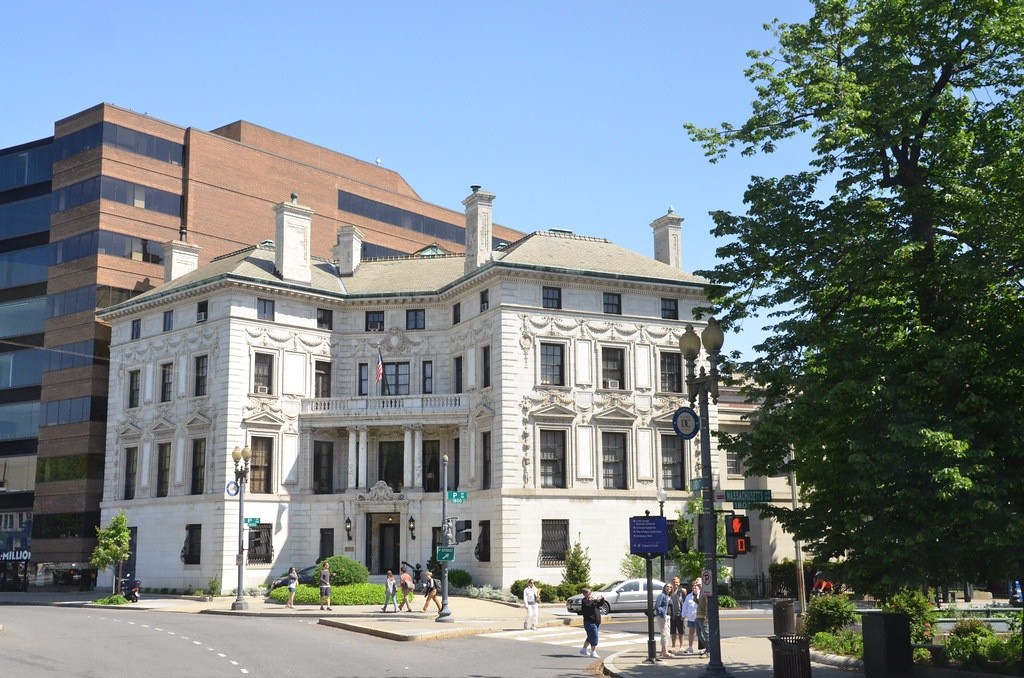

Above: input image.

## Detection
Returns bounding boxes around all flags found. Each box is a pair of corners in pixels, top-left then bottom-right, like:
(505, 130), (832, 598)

(375, 351), (383, 385)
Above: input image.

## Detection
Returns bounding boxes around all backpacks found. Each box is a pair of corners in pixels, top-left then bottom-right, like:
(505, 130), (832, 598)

(434, 579), (442, 592)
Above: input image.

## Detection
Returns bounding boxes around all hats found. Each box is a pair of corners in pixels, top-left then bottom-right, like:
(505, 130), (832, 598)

(582, 589), (590, 596)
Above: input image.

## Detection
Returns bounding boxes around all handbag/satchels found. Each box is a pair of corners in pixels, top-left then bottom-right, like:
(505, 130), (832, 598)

(535, 597), (540, 604)
(393, 583), (398, 593)
(403, 580), (415, 590)
(644, 609), (656, 618)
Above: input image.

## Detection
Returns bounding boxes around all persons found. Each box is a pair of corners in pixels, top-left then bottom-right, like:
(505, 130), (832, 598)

(524, 579), (538, 631)
(681, 577), (710, 659)
(380, 570), (400, 613)
(813, 578), (833, 592)
(668, 578), (687, 652)
(285, 566), (299, 608)
(319, 561), (332, 611)
(418, 571), (442, 613)
(656, 583), (675, 658)
(398, 567), (413, 612)
(580, 588), (605, 659)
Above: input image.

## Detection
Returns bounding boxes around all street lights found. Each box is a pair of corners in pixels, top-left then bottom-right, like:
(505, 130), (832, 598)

(231, 442), (253, 611)
(655, 487), (668, 583)
(678, 315), (736, 678)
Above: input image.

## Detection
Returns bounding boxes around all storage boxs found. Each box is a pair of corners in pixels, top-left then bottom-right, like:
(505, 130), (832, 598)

(132, 580), (141, 587)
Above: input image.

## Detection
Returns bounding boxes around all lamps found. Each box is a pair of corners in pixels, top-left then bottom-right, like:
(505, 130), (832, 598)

(387, 516), (393, 522)
(346, 516), (353, 540)
(409, 516), (416, 541)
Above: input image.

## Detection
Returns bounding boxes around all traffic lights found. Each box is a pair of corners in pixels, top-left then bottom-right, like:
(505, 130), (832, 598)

(724, 515), (752, 555)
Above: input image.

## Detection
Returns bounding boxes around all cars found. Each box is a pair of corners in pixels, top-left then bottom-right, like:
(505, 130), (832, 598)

(566, 577), (668, 617)
(4, 566), (83, 592)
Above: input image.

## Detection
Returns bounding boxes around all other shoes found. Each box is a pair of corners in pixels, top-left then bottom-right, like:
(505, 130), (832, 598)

(669, 648), (676, 652)
(327, 607), (332, 611)
(420, 610), (426, 612)
(684, 648), (693, 653)
(679, 648), (683, 652)
(320, 607), (324, 610)
(580, 647), (590, 657)
(287, 604), (295, 609)
(381, 608), (386, 612)
(530, 624), (537, 630)
(524, 622), (527, 629)
(590, 652), (600, 658)
(699, 654), (710, 659)
(698, 649), (706, 654)
(407, 610), (412, 612)
(659, 652), (673, 658)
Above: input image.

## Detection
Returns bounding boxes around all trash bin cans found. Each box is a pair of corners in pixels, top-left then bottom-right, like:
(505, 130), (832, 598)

(1009, 581), (1024, 604)
(772, 598), (797, 635)
(767, 635), (814, 678)
(864, 612), (913, 678)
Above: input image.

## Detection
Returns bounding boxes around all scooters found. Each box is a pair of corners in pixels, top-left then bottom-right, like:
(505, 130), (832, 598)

(115, 572), (142, 603)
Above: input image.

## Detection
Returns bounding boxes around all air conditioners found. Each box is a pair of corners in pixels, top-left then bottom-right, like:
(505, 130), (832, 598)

(481, 303), (487, 310)
(368, 321), (381, 331)
(606, 379), (620, 389)
(256, 385), (268, 394)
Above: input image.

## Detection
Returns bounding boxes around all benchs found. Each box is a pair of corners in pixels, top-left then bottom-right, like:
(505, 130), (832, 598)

(909, 642), (948, 665)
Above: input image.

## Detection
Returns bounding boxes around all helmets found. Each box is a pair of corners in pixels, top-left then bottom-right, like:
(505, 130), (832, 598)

(815, 571), (822, 576)
